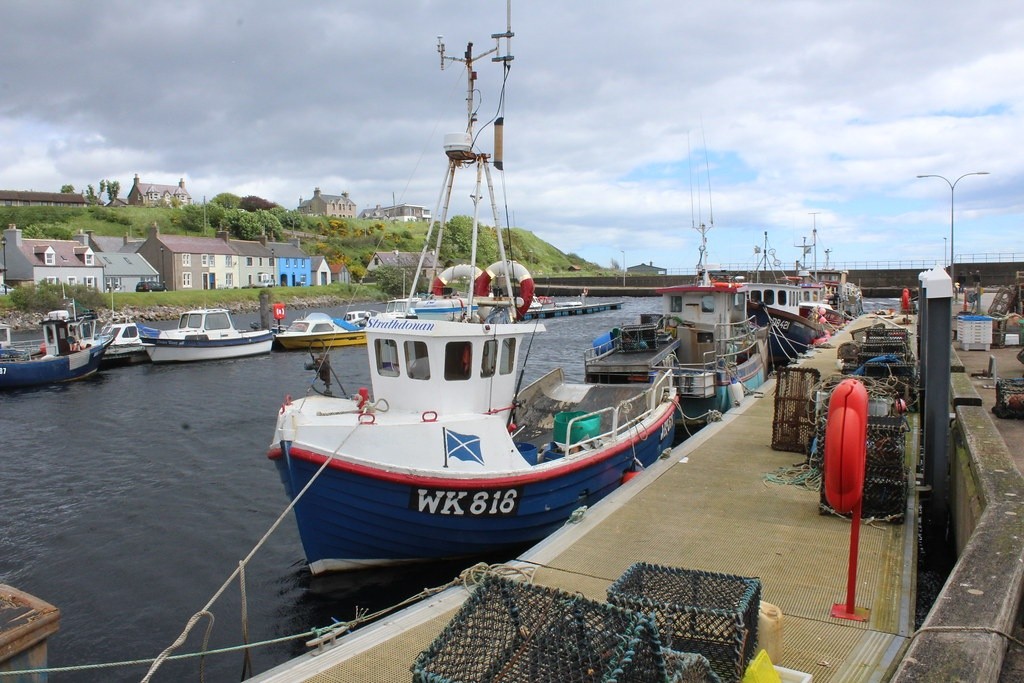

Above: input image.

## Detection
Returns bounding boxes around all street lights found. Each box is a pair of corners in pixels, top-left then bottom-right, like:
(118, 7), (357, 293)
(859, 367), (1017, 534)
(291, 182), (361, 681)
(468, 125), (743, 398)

(916, 172), (991, 293)
(160, 245), (166, 288)
(620, 250), (626, 287)
(1, 236), (8, 295)
(943, 237), (947, 272)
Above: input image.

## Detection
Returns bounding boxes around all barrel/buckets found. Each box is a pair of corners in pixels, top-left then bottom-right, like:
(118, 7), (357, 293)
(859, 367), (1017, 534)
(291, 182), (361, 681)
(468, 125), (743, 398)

(552, 410), (602, 444)
(756, 601), (784, 666)
(592, 327), (622, 356)
(513, 442), (538, 466)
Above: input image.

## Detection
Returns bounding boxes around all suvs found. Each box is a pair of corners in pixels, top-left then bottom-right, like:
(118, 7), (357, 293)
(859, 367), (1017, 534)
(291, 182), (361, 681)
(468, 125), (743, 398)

(136, 281), (168, 292)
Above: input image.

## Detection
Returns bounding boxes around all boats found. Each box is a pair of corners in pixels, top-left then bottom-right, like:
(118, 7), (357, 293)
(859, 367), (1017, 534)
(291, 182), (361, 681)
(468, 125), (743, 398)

(0, 324), (11, 350)
(135, 290), (276, 366)
(78, 315), (148, 362)
(579, 113), (771, 428)
(0, 281), (115, 394)
(552, 301), (582, 307)
(266, 0), (683, 580)
(696, 230), (865, 374)
(537, 296), (554, 305)
(518, 296), (542, 309)
(341, 310), (371, 327)
(273, 306), (370, 349)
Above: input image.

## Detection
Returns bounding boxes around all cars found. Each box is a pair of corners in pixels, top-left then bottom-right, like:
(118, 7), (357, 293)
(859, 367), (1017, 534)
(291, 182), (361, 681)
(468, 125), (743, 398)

(0, 284), (16, 295)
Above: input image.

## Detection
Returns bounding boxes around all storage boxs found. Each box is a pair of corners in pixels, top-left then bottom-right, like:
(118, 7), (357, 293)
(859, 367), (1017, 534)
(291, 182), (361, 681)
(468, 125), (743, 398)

(750, 659), (812, 683)
(957, 315), (993, 351)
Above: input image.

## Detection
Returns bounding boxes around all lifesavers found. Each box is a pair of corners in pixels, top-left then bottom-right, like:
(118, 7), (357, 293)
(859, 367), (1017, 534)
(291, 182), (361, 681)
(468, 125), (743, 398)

(824, 377), (869, 517)
(902, 288), (909, 309)
(475, 260), (535, 323)
(432, 263), (490, 322)
(713, 282), (742, 288)
(781, 276), (803, 280)
